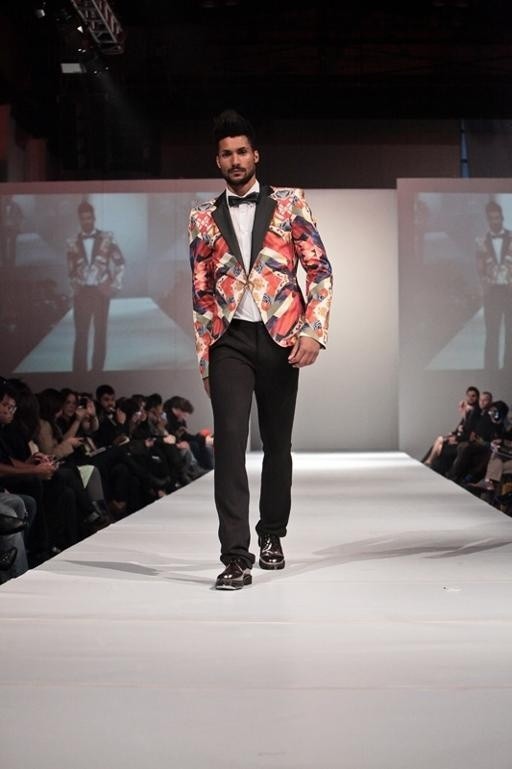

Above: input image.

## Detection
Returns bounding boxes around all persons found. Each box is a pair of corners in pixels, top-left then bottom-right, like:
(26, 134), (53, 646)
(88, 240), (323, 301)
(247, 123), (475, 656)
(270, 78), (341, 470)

(418, 381), (512, 518)
(468, 198), (511, 372)
(181, 120), (336, 589)
(61, 199), (126, 374)
(0, 371), (212, 589)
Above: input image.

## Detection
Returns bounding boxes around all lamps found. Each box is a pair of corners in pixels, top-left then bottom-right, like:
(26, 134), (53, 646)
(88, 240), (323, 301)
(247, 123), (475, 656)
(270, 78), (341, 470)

(36, 1), (113, 79)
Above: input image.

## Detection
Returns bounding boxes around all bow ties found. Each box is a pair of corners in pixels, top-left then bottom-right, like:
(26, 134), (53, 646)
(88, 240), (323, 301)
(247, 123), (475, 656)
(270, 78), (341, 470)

(228, 191), (258, 206)
(83, 234), (94, 239)
(492, 233), (502, 238)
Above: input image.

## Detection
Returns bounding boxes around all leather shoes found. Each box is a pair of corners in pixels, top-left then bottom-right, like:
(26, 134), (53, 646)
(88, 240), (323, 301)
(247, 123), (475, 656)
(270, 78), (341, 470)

(258, 535), (285, 570)
(215, 562), (251, 590)
